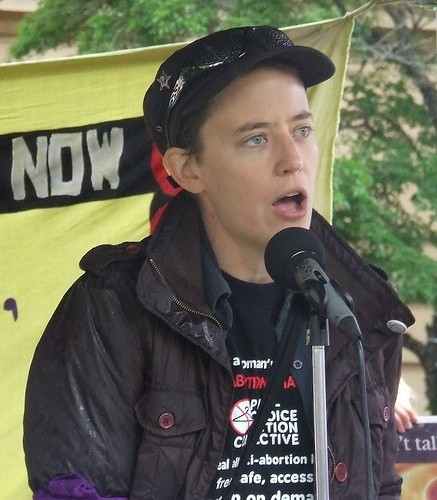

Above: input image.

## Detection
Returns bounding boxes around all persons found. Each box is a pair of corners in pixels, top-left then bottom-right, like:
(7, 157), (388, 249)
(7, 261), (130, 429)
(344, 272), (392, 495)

(21, 28), (415, 499)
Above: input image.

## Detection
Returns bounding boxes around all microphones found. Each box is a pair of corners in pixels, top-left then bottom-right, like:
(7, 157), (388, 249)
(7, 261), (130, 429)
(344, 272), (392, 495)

(263, 227), (361, 344)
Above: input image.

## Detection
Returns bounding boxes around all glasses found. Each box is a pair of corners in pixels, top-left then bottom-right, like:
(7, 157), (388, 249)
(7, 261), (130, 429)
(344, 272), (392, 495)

(166, 25), (295, 149)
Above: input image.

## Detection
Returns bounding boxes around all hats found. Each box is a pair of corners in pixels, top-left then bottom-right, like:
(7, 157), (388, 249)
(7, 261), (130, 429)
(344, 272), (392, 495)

(144, 25), (336, 157)
